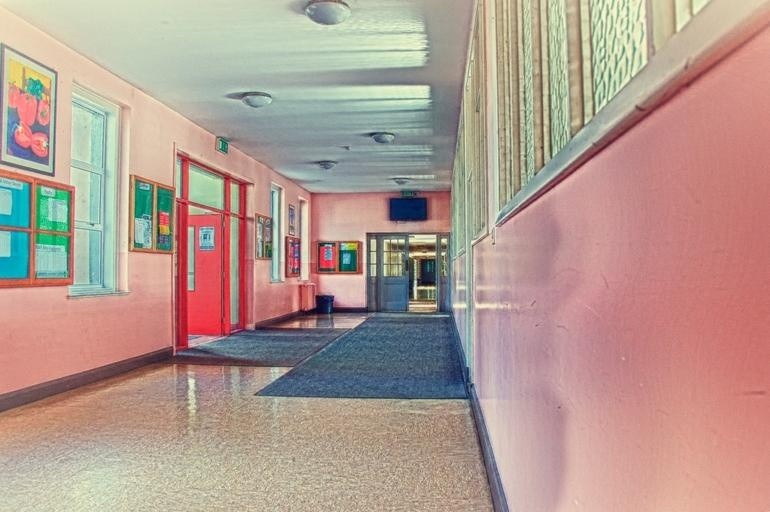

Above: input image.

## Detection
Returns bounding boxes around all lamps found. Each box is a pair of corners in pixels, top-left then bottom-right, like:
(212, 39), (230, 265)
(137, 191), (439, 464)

(240, 92), (272, 108)
(318, 161), (339, 170)
(304, 0), (352, 26)
(370, 132), (396, 143)
(393, 177), (411, 185)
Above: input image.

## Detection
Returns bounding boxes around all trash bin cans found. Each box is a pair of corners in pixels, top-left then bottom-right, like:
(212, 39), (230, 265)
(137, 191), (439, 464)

(316, 295), (334, 314)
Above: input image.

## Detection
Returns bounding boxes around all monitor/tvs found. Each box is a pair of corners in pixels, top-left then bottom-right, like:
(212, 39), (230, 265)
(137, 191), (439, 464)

(389, 198), (427, 221)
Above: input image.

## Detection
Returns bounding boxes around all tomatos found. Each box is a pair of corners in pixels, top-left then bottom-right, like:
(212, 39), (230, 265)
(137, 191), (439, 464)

(7, 82), (52, 158)
